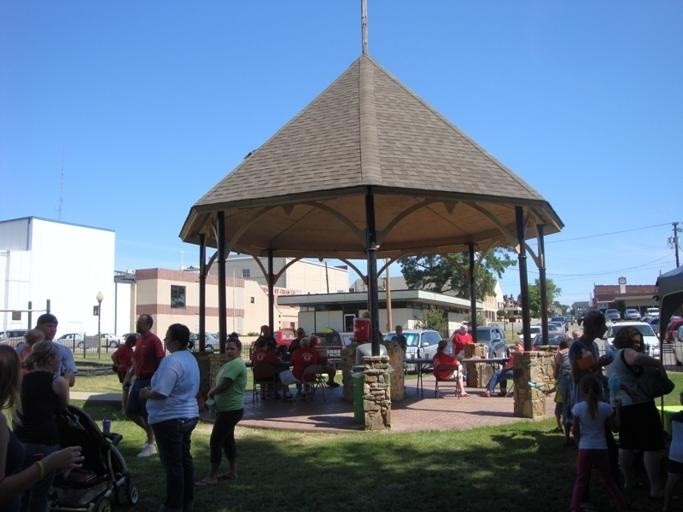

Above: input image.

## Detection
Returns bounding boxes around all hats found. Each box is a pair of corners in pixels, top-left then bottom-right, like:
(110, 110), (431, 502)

(606, 309), (621, 319)
(56, 333), (84, 349)
(624, 309), (641, 320)
(532, 332), (567, 349)
(95, 333), (120, 348)
(119, 333), (136, 345)
(548, 324), (560, 335)
(194, 332), (218, 353)
(644, 307), (659, 316)
(517, 325), (542, 344)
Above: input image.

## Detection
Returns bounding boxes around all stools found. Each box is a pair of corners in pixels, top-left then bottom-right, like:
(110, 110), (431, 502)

(136, 374), (149, 381)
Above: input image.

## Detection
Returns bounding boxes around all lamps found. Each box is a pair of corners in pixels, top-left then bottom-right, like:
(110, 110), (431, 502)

(253, 361), (290, 400)
(300, 363), (326, 401)
(435, 364), (460, 399)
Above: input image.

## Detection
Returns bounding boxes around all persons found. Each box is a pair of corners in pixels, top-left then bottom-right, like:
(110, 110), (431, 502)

(0, 343), (85, 512)
(608, 325), (666, 497)
(453, 325), (473, 359)
(432, 340), (471, 396)
(250, 337), (279, 400)
(12, 339), (75, 447)
(19, 329), (45, 376)
(568, 330), (582, 347)
(279, 336), (320, 400)
(391, 325), (407, 352)
(15, 314), (77, 387)
(124, 313), (165, 458)
(481, 343), (524, 397)
(569, 375), (625, 512)
(568, 311), (613, 383)
(289, 328), (306, 354)
(111, 334), (136, 415)
(138, 323), (200, 512)
(309, 335), (340, 387)
(260, 325), (277, 349)
(661, 391), (683, 512)
(551, 341), (573, 438)
(194, 332), (247, 487)
(544, 377), (565, 433)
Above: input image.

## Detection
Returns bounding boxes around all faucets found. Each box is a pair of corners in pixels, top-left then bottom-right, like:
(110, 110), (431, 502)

(460, 393), (470, 397)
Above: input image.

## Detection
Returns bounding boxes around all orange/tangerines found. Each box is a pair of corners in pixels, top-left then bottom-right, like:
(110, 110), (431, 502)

(46, 405), (141, 512)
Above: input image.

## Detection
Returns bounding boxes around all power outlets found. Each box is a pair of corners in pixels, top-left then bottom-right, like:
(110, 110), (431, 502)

(312, 331), (355, 361)
(250, 327), (298, 362)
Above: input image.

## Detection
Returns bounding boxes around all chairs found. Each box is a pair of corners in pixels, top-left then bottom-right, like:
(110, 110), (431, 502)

(326, 381), (340, 387)
(136, 444), (158, 458)
(482, 391), (490, 397)
(285, 394), (293, 399)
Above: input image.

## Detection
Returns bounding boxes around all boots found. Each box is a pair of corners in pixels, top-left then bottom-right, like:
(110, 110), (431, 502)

(384, 330), (442, 372)
(468, 326), (507, 359)
(604, 322), (661, 360)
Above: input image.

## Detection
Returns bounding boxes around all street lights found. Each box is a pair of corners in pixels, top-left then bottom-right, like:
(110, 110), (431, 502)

(621, 348), (675, 400)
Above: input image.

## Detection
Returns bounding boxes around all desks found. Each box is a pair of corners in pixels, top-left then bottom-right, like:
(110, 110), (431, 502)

(94, 290), (106, 362)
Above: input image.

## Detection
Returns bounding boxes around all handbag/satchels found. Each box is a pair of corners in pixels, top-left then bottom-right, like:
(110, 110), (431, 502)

(35, 461), (45, 480)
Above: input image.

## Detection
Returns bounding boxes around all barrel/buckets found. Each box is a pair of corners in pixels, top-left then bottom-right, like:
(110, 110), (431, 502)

(193, 478), (218, 487)
(649, 491), (679, 503)
(217, 471), (240, 481)
